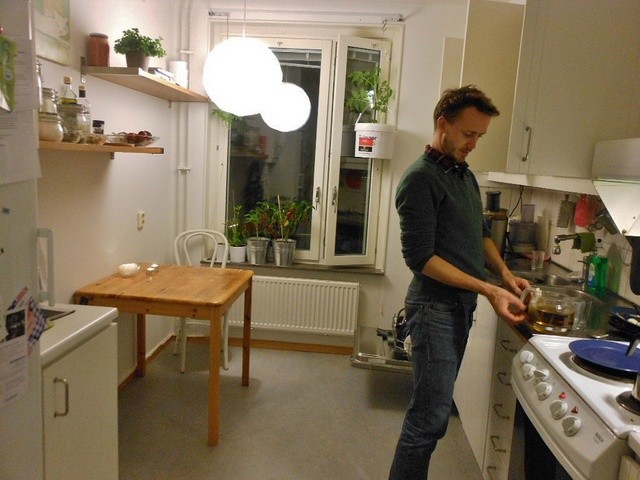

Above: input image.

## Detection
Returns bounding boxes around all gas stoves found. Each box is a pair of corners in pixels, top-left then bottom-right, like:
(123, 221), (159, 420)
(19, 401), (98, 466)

(506, 335), (640, 443)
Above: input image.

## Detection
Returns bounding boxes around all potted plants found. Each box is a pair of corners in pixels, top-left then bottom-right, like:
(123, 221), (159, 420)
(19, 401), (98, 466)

(258, 201), (316, 267)
(344, 63), (397, 158)
(221, 205), (246, 263)
(113, 27), (168, 72)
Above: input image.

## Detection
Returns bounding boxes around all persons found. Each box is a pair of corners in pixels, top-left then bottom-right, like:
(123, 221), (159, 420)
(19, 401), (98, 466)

(387, 84), (533, 480)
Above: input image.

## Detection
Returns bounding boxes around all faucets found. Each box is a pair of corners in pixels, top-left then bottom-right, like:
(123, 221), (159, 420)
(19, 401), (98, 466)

(552, 234), (579, 254)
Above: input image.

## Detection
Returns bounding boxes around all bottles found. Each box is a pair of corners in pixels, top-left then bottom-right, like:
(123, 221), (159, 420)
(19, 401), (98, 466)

(41, 87), (59, 116)
(58, 76), (78, 143)
(35, 59), (45, 114)
(92, 120), (104, 144)
(76, 84), (92, 144)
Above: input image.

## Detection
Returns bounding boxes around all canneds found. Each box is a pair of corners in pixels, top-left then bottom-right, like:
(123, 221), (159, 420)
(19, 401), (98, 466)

(92, 120), (105, 134)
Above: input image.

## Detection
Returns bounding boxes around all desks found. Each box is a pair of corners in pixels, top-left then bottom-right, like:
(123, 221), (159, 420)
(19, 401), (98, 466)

(73, 263), (254, 446)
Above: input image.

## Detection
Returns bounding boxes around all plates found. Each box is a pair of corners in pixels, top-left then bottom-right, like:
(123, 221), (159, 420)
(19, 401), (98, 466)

(105, 132), (160, 147)
(609, 304), (640, 331)
(569, 339), (640, 375)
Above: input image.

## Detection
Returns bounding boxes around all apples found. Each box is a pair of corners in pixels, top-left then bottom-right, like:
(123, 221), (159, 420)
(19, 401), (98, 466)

(138, 131), (147, 142)
(145, 131), (152, 141)
(127, 132), (137, 143)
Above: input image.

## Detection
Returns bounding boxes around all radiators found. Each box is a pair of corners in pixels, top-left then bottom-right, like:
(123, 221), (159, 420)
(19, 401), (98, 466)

(182, 274), (360, 359)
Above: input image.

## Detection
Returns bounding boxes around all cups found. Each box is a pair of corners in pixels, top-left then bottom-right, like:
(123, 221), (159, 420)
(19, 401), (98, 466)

(568, 296), (593, 333)
(530, 250), (545, 270)
(151, 263), (159, 272)
(146, 267), (155, 275)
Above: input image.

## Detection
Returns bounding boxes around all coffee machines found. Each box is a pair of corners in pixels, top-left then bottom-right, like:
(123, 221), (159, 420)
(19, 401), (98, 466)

(480, 190), (509, 264)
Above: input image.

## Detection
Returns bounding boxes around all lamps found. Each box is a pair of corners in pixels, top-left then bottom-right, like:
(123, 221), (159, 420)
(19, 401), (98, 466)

(203, 1), (282, 116)
(259, 67), (311, 134)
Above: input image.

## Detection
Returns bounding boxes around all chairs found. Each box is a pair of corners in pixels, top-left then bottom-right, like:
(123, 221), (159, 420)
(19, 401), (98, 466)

(172, 229), (231, 373)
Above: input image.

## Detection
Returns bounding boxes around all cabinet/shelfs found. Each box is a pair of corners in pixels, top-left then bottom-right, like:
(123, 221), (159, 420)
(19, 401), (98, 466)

(41, 301), (119, 480)
(439, 36), (463, 99)
(0, 0), (45, 479)
(39, 66), (208, 160)
(481, 318), (572, 480)
(617, 433), (640, 480)
(459, 0), (640, 197)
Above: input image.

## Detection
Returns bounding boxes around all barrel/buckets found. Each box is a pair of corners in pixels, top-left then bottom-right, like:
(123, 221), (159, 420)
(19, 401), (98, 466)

(248, 236), (270, 265)
(341, 124), (355, 157)
(272, 238), (297, 267)
(354, 101), (397, 159)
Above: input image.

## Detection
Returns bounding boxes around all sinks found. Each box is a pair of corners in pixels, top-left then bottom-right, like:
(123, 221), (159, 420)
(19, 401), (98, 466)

(494, 270), (572, 289)
(553, 289), (603, 305)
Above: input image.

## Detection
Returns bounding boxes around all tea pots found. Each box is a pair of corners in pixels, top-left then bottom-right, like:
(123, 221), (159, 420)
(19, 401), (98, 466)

(118, 262), (141, 278)
(519, 287), (576, 337)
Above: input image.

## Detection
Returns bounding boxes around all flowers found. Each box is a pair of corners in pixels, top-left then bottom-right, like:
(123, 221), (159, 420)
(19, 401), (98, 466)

(242, 208), (270, 265)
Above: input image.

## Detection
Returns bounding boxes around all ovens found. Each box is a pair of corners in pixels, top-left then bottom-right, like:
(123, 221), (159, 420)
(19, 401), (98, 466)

(500, 377), (589, 480)
